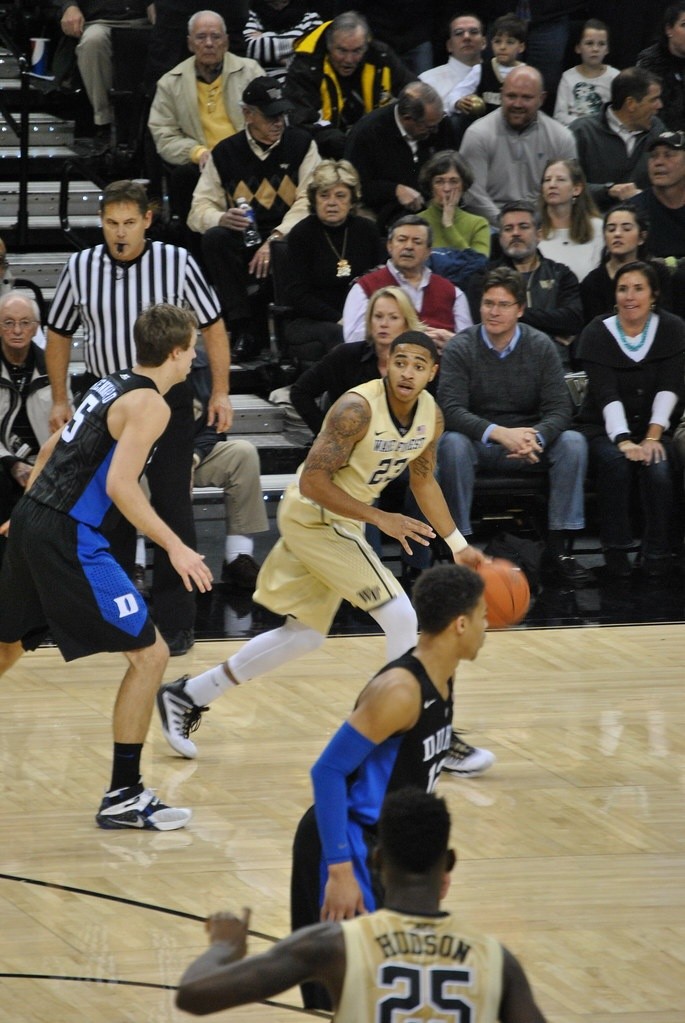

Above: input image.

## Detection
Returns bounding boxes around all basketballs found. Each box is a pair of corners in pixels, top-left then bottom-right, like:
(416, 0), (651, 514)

(472, 557), (531, 627)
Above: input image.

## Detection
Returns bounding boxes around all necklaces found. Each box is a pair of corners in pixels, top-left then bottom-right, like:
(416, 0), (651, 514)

(324, 224), (351, 277)
(615, 312), (652, 351)
(552, 226), (572, 244)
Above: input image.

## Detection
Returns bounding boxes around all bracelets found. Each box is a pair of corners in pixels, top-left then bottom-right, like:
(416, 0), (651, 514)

(194, 148), (208, 165)
(646, 437), (661, 442)
(267, 235), (281, 241)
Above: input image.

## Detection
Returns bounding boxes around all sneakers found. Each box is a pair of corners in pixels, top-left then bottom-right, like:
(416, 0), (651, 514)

(157, 674), (211, 760)
(551, 552), (588, 579)
(221, 553), (261, 590)
(442, 729), (497, 777)
(98, 774), (191, 832)
(281, 353), (302, 372)
(133, 567), (151, 598)
(161, 625), (195, 656)
(228, 337), (271, 371)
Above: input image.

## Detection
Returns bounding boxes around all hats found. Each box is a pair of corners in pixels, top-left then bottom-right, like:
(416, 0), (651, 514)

(646, 131), (685, 150)
(244, 76), (295, 116)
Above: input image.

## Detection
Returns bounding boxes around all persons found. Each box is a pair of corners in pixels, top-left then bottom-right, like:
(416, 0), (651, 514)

(0, 0), (685, 654)
(176, 793), (549, 1023)
(157, 330), (496, 777)
(289, 566), (490, 1011)
(0, 303), (214, 832)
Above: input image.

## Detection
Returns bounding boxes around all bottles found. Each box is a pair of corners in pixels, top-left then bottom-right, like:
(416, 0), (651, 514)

(237, 197), (262, 247)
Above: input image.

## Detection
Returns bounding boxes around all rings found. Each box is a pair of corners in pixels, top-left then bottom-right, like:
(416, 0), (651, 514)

(264, 260), (269, 264)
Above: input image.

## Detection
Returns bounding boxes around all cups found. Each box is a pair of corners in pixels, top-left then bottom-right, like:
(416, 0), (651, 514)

(29, 37), (50, 76)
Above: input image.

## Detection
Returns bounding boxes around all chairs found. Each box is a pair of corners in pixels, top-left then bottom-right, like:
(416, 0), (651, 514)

(0, 0), (684, 561)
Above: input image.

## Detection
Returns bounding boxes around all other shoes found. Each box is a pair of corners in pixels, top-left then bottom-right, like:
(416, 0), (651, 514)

(80, 137), (118, 172)
(607, 559), (632, 578)
(644, 557), (672, 576)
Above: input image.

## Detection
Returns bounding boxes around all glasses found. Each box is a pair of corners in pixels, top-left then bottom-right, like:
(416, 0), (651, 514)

(4, 320), (39, 328)
(484, 299), (519, 310)
(433, 180), (460, 189)
